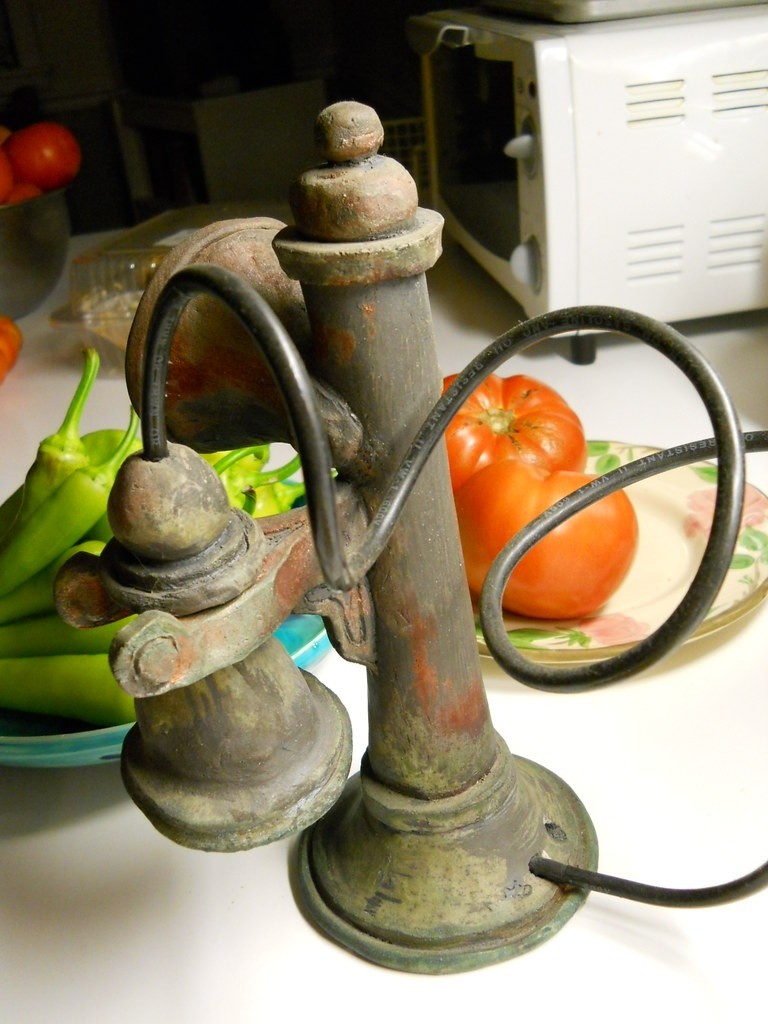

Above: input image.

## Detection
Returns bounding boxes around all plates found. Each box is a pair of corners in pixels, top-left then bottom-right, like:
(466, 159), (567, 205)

(2, 616), (335, 771)
(468, 440), (768, 665)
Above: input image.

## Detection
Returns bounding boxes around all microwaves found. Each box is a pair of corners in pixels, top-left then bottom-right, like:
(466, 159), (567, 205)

(398, 6), (768, 365)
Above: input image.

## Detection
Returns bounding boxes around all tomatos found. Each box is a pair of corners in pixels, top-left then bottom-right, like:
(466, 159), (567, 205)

(438, 374), (637, 620)
(0, 121), (81, 205)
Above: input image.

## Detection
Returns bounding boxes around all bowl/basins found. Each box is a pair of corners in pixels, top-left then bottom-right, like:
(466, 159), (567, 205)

(1, 189), (72, 323)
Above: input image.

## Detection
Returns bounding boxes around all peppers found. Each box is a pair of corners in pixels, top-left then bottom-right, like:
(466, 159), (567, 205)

(0, 350), (310, 722)
